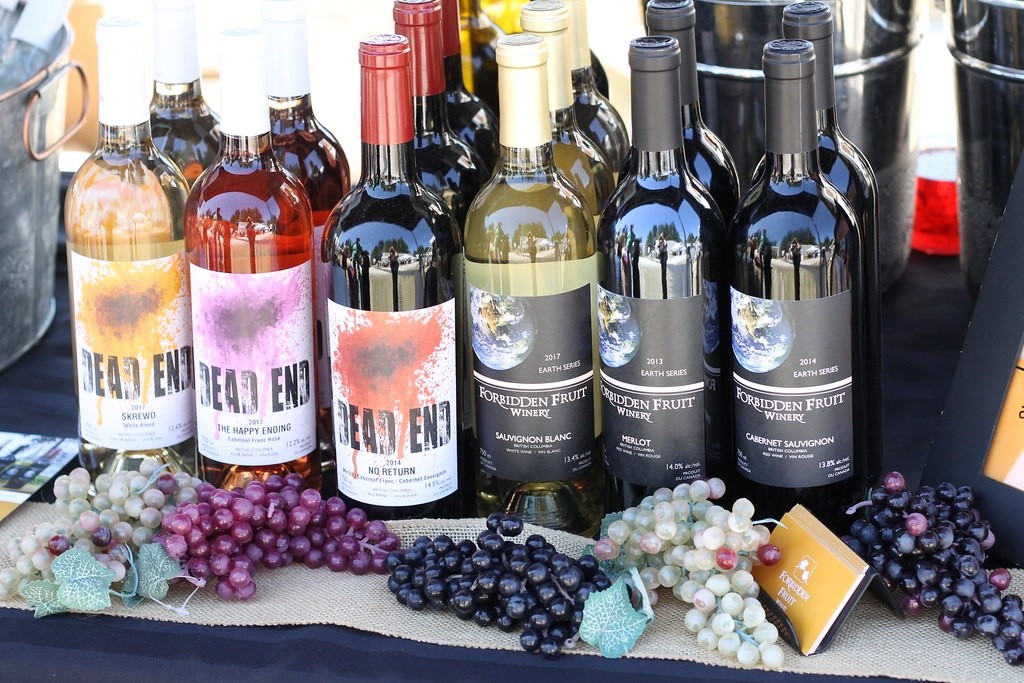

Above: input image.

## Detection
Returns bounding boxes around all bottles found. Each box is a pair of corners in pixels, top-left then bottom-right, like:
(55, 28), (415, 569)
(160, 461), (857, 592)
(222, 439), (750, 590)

(389, 0), (501, 237)
(640, 0), (741, 227)
(185, 31), (318, 491)
(465, 33), (601, 536)
(517, 0), (630, 235)
(920, 153), (1024, 569)
(259, 3), (352, 420)
(596, 35), (729, 515)
(779, 0), (877, 489)
(729, 38), (864, 539)
(321, 34), (479, 523)
(150, 0), (223, 187)
(66, 18), (196, 478)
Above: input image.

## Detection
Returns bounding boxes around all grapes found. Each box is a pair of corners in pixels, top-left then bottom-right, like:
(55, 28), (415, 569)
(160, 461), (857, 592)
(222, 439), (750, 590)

(0, 458), (1024, 671)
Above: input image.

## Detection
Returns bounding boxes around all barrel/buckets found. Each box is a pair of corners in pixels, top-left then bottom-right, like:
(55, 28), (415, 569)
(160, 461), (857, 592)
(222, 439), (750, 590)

(0, 0), (90, 369)
(947, 0), (1023, 307)
(638, 0), (921, 296)
